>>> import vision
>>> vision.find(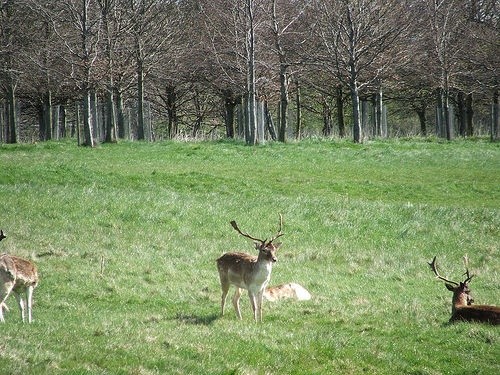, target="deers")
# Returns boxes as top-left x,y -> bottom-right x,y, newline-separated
426,253 -> 500,327
215,212 -> 286,325
0,229 -> 39,324
262,282 -> 311,303
